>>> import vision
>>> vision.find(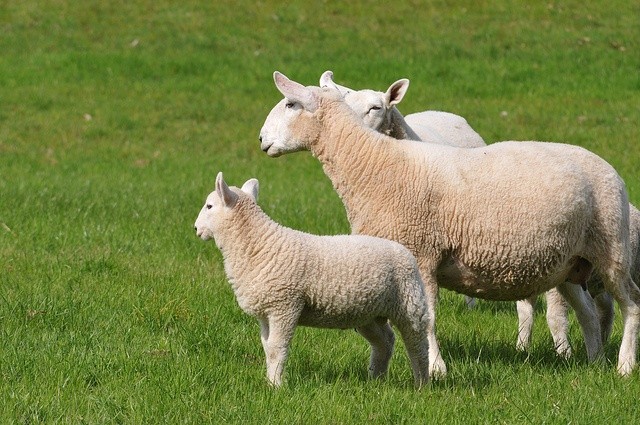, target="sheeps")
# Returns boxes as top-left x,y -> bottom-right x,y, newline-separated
515,201 -> 640,361
333,78 -> 487,310
257,70 -> 640,378
192,171 -> 430,391
